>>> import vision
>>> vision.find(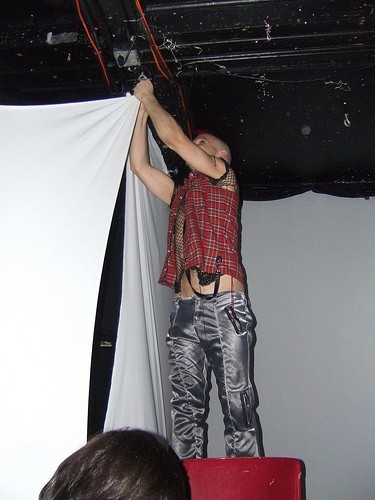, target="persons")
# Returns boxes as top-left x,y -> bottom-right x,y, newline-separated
128,78 -> 261,459
38,427 -> 192,500
